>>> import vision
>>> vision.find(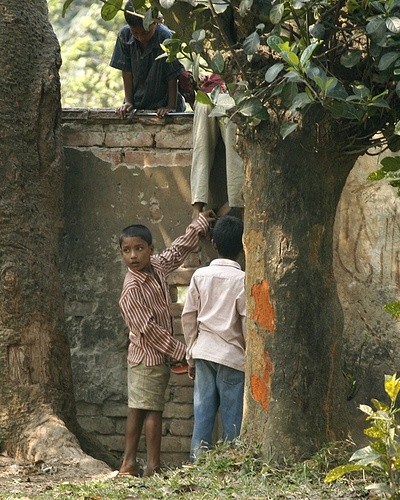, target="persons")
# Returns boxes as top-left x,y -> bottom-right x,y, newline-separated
119,209 -> 218,478
109,0 -> 187,119
178,68 -> 246,240
180,216 -> 246,464
